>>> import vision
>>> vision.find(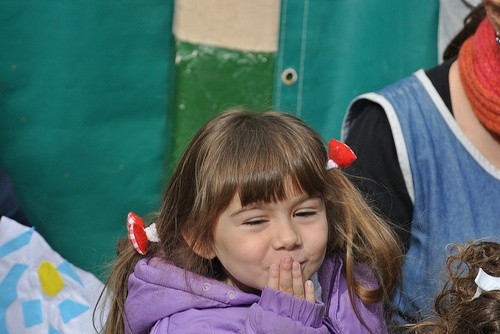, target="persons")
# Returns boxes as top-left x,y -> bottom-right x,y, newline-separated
93,104 -> 406,334
341,1 -> 500,334
401,239 -> 500,334
